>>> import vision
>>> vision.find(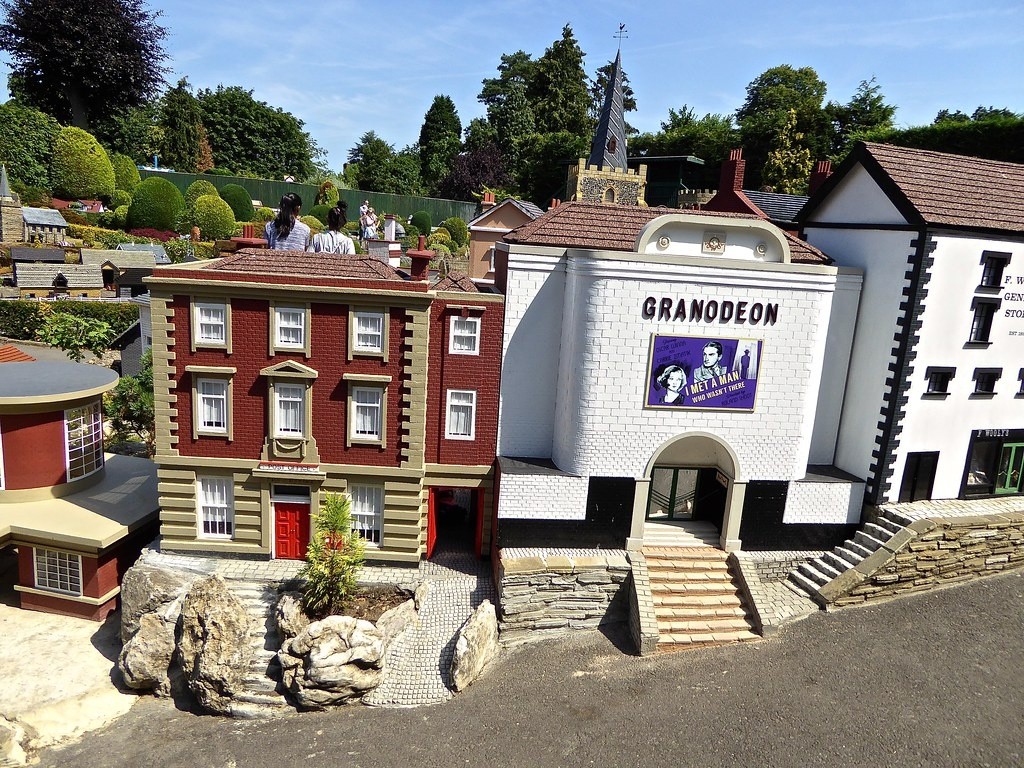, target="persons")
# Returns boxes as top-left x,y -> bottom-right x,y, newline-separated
264,191 -> 310,253
358,200 -> 380,250
311,207 -> 356,255
